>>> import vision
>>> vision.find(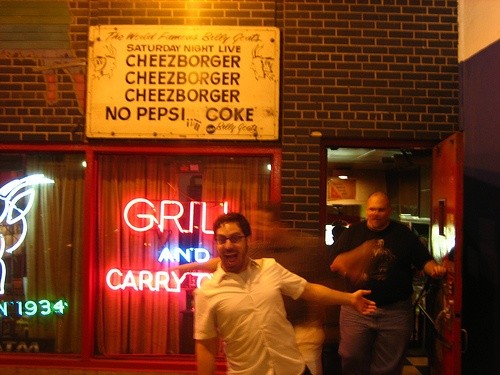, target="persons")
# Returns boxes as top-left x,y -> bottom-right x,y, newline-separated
324,191 -> 447,375
192,213 -> 377,375
